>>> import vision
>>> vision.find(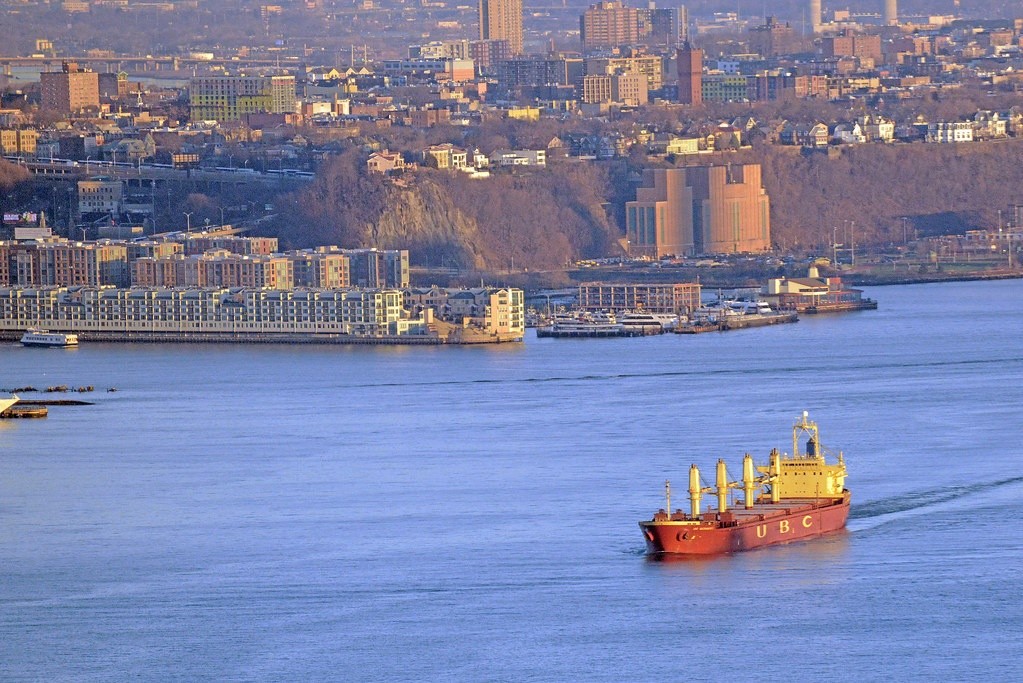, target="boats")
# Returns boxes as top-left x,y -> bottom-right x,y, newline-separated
637,410 -> 852,555
19,327 -> 79,347
